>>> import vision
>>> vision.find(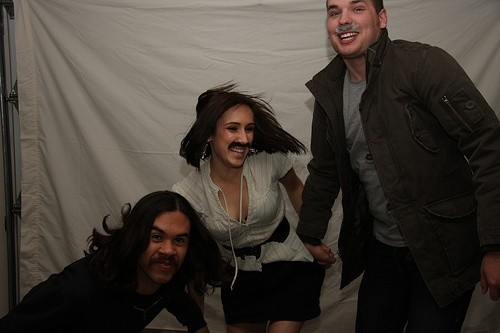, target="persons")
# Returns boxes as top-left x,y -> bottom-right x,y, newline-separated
0,190 -> 223,333
295,1 -> 500,333
171,82 -> 336,333
